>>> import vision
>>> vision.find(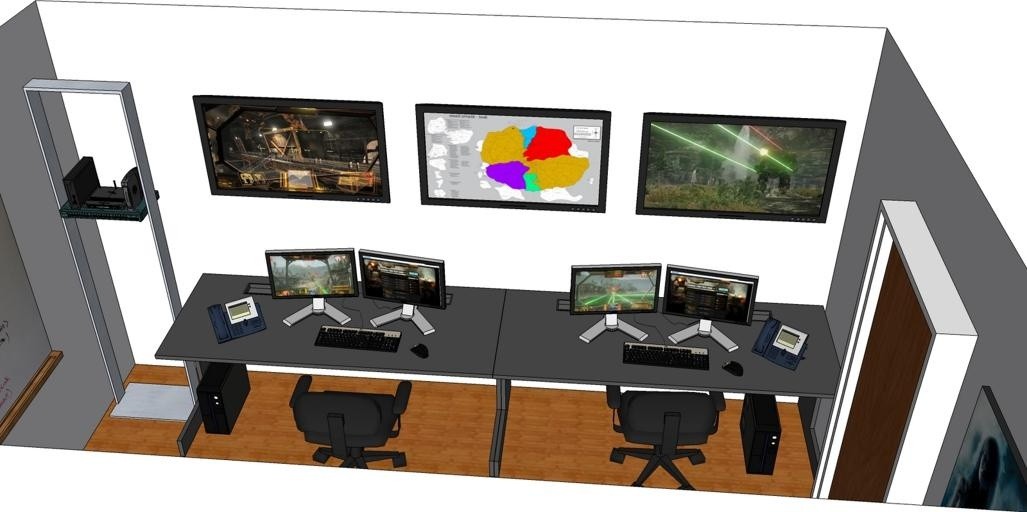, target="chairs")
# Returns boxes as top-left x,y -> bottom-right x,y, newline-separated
606,386 -> 728,491
